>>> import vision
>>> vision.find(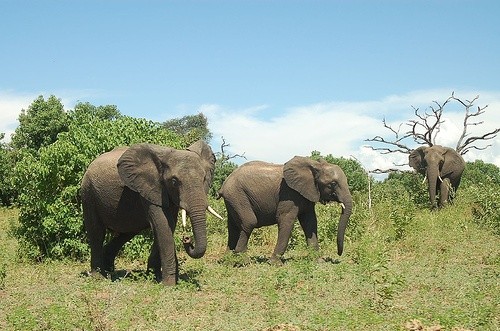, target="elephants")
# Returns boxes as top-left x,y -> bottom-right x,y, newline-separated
409,145 -> 466,209
76,139 -> 223,288
215,156 -> 352,266
